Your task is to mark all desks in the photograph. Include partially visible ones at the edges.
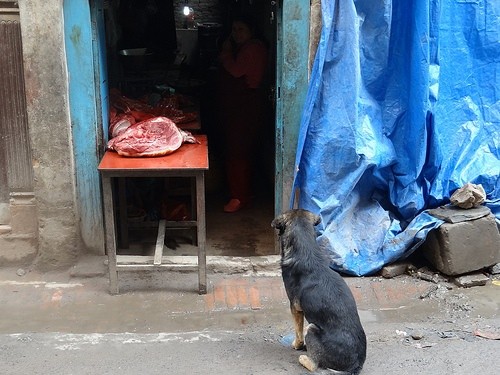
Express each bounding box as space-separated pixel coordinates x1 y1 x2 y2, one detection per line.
97 134 210 295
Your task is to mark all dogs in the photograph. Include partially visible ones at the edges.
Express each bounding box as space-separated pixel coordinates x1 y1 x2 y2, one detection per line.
270 209 367 375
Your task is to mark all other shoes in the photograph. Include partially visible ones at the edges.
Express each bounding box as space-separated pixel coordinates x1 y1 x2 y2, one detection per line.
224 197 244 212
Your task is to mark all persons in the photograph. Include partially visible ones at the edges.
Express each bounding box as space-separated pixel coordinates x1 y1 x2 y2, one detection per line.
218 15 269 213
